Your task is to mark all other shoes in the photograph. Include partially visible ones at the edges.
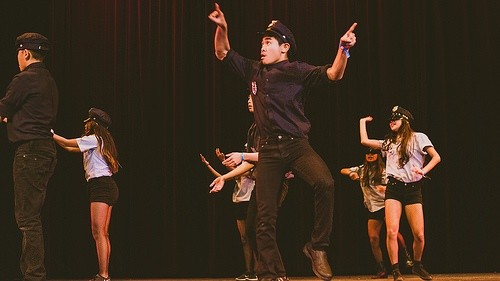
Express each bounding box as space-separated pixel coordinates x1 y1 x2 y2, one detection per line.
261 276 290 281
393 270 403 281
371 272 388 279
236 272 259 281
302 243 333 281
406 261 414 274
88 273 111 281
412 263 433 280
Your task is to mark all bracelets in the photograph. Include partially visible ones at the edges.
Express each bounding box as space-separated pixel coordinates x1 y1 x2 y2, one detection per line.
51 132 54 136
241 152 244 160
349 171 353 176
339 44 350 58
421 170 424 176
205 163 209 166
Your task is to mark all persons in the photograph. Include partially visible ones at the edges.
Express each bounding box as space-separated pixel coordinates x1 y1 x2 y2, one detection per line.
208 3 358 281
199 106 441 281
51 106 121 281
0 33 58 281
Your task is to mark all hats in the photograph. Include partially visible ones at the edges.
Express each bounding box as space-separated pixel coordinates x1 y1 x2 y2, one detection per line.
390 105 414 121
15 32 49 51
257 19 296 47
84 107 112 130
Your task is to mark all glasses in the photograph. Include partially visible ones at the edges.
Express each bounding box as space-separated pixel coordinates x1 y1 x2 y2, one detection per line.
365 149 381 154
